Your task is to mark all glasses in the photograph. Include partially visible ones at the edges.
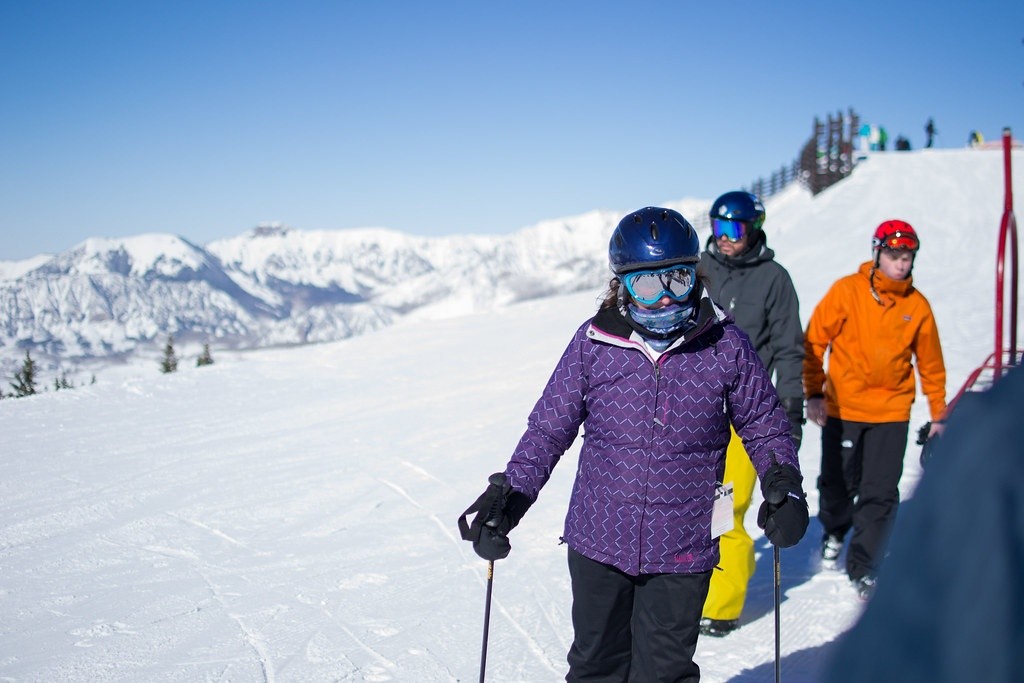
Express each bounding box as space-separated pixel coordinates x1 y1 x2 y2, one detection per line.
883 231 919 250
616 264 696 308
711 217 755 243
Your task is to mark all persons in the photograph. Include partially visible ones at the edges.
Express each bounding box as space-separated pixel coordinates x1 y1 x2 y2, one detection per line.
694 191 807 637
803 219 947 602
461 206 810 683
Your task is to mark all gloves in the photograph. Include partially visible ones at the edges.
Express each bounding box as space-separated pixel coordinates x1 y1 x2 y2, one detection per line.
757 463 809 548
780 398 805 451
916 422 941 468
458 483 531 560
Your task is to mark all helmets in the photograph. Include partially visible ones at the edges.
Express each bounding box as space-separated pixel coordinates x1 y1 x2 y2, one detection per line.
872 220 919 252
709 191 765 235
609 207 699 274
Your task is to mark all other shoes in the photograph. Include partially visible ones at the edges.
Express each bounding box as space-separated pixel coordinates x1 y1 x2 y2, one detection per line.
698 617 739 637
822 535 843 569
848 571 881 601
